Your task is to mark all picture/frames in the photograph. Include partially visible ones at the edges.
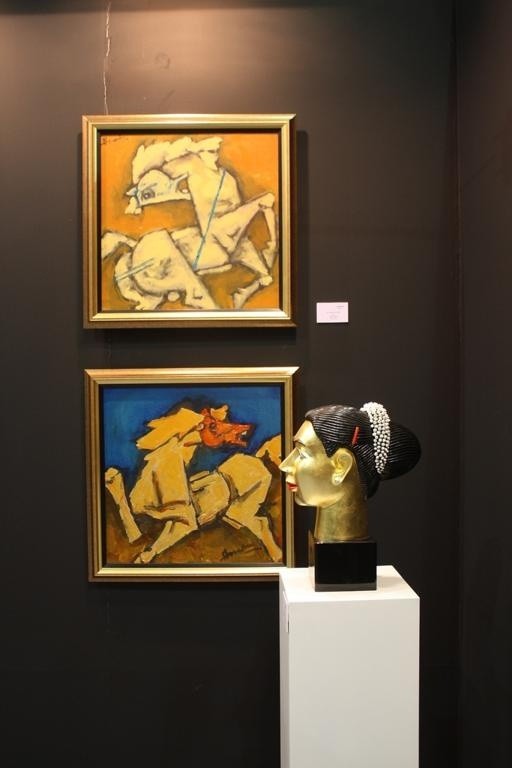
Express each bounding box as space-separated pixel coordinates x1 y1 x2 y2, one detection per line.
83 368 296 584
80 113 298 333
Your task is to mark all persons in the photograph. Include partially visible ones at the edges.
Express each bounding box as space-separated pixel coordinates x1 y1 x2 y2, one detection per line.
276 396 423 542
